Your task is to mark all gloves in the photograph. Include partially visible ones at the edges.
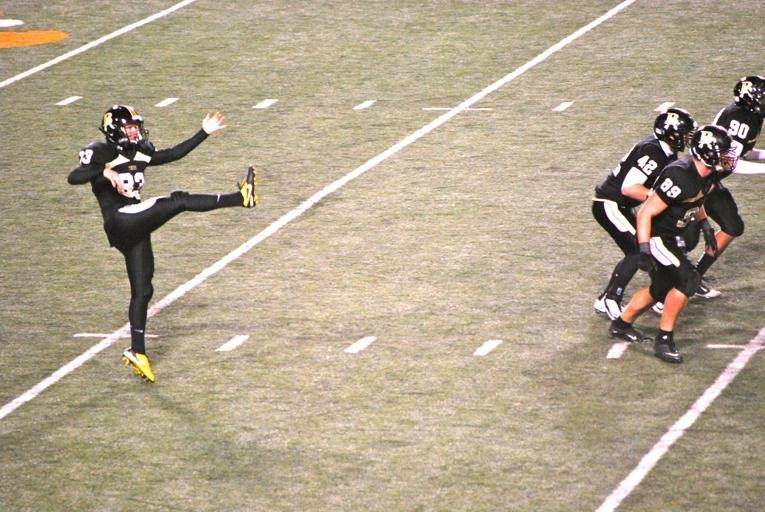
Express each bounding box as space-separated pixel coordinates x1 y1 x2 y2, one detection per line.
702 227 718 258
638 252 659 274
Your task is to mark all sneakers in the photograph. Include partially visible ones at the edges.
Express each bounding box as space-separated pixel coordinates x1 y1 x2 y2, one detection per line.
238 164 259 208
653 336 683 363
607 319 653 343
648 300 665 316
593 292 621 321
121 346 154 384
694 283 722 299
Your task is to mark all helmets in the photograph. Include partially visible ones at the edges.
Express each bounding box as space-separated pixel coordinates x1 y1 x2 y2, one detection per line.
654 108 700 152
734 75 765 121
99 105 149 154
690 124 738 173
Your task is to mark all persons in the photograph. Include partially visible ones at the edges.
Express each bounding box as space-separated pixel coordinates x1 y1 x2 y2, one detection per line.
593 77 764 363
68 104 257 382
